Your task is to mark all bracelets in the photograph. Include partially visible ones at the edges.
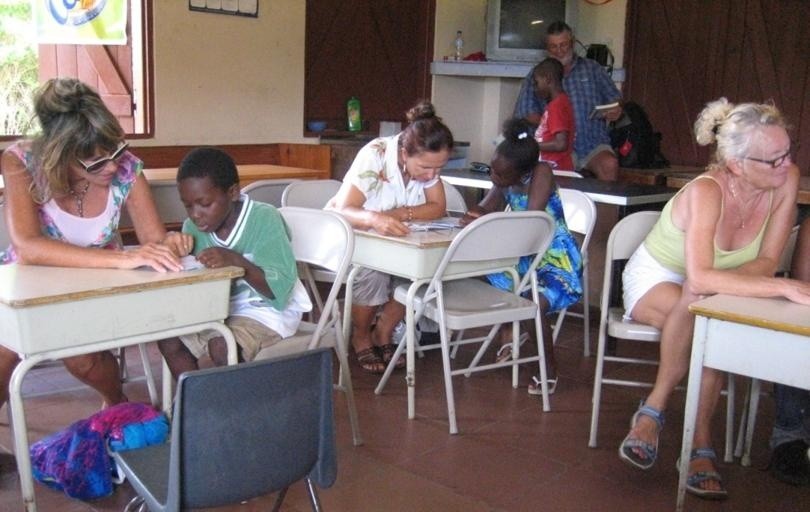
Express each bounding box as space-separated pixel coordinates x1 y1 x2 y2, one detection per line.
403 205 413 221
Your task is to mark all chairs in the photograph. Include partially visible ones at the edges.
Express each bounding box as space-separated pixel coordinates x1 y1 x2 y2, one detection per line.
449 186 596 388
588 211 737 462
373 209 558 435
282 178 424 359
237 177 316 323
106 346 338 511
250 205 364 447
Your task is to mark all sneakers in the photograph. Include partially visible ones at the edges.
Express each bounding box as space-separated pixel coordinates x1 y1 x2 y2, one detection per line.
770 439 809 486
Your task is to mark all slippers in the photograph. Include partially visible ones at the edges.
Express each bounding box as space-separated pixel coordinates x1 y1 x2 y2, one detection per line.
528 374 558 395
675 448 727 500
497 333 528 363
351 342 406 373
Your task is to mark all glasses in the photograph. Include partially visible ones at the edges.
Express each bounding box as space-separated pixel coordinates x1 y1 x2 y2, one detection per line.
74 140 128 172
743 141 798 168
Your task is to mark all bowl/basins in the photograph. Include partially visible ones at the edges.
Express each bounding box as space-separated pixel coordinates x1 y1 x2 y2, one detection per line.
307 121 328 134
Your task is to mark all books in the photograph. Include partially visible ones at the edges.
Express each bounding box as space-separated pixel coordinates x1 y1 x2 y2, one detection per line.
589 102 619 121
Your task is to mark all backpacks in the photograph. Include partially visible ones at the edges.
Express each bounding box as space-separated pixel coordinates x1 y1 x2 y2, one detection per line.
608 101 670 169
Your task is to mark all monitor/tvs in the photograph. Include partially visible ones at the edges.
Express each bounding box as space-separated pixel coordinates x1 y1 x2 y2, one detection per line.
486 0 577 65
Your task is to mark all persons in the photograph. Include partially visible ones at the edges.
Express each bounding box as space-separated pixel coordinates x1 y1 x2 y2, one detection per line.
1 79 195 411
322 100 452 374
619 96 809 499
512 20 624 182
769 217 810 486
458 118 583 391
157 147 313 382
533 57 574 171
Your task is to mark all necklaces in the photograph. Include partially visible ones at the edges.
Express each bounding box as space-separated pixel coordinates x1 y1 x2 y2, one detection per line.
65 180 90 217
729 180 758 229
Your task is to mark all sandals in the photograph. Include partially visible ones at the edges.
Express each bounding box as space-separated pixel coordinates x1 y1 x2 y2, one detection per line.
620 402 665 470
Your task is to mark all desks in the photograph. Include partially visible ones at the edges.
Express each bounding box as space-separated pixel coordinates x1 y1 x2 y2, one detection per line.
320 138 471 181
1 243 245 512
339 217 520 420
675 295 810 512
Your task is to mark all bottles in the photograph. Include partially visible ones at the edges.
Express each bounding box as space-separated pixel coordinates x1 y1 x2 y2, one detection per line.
347 95 362 132
454 30 464 61
